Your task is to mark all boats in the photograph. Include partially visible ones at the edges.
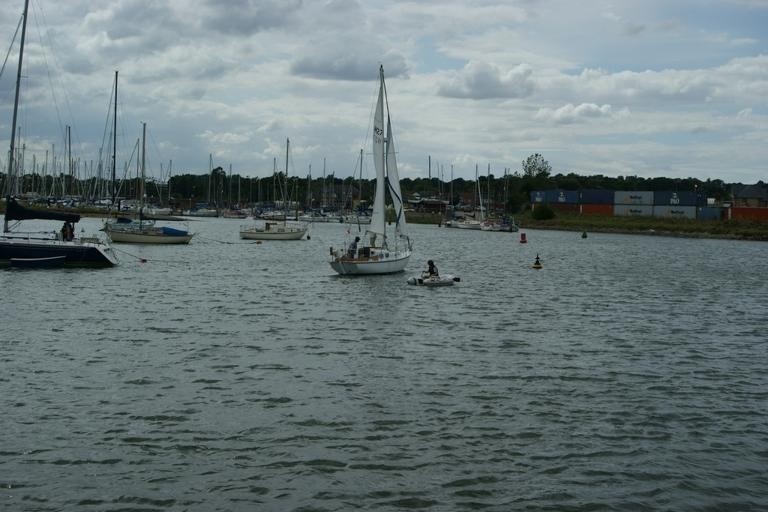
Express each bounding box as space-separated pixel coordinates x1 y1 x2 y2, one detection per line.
403 270 461 290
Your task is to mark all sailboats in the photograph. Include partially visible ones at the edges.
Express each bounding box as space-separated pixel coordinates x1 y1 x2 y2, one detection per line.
99 121 194 244
0 0 123 268
236 136 309 240
194 153 220 218
249 137 520 242
0 72 187 221
324 62 416 277
222 174 248 220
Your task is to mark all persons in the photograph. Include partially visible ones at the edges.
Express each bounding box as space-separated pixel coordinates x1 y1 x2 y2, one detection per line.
347 235 361 259
421 258 438 278
